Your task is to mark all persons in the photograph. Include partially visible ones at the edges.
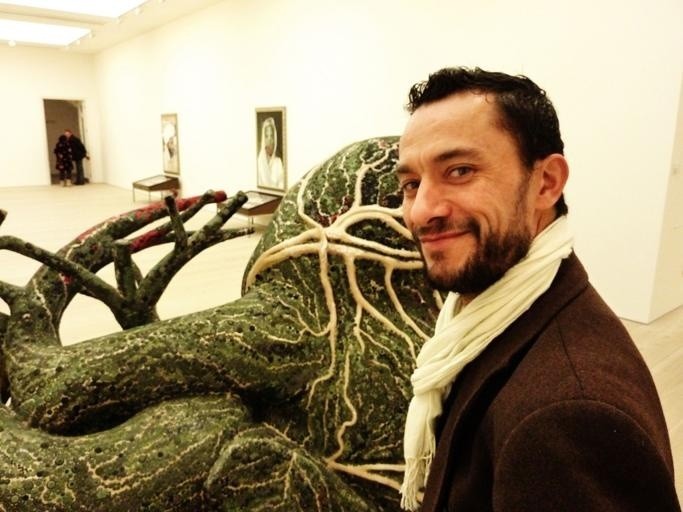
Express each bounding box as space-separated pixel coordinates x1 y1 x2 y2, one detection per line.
64 129 87 185
393 67 681 512
53 133 74 186
256 117 284 191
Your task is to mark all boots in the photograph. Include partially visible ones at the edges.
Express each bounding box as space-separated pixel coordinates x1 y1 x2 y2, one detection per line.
59 179 72 187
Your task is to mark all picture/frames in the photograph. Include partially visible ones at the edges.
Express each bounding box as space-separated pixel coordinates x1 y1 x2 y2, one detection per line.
252 106 288 194
159 113 181 176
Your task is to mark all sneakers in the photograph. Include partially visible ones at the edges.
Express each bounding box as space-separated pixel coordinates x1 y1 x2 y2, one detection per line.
73 180 83 185
